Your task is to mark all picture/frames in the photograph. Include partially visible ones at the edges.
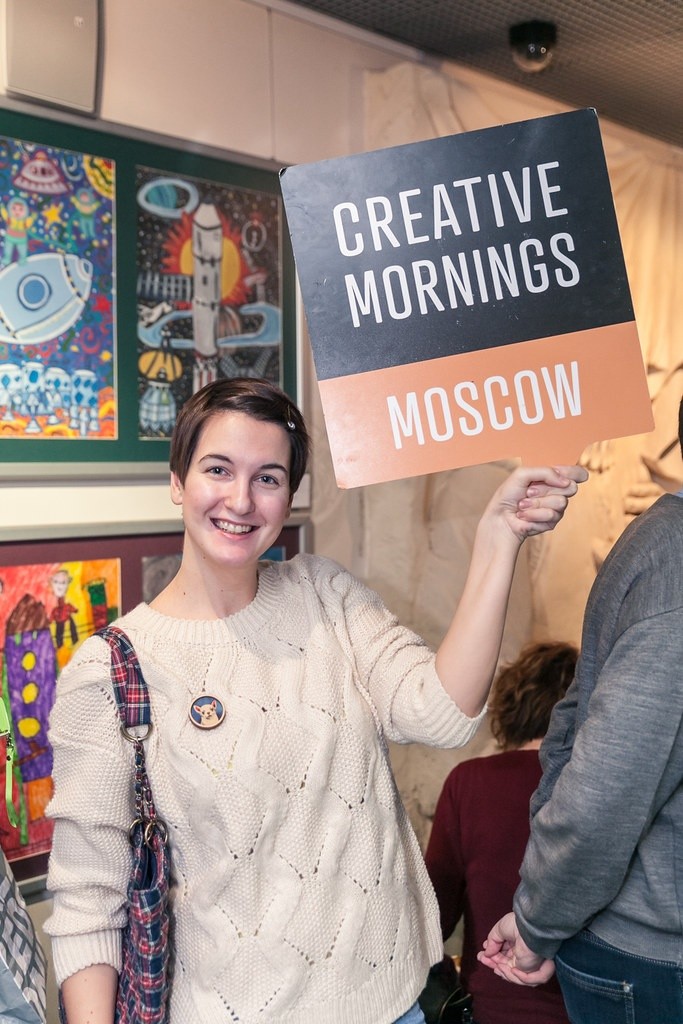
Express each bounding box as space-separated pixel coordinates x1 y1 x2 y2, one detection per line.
0 94 306 484
0 511 315 899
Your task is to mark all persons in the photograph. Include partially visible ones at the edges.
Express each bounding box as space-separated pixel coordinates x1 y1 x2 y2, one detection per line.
477 392 683 1024
425 640 582 1024
43 376 588 1024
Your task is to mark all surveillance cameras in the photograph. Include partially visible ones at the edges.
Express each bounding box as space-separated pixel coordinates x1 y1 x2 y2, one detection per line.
507 20 557 72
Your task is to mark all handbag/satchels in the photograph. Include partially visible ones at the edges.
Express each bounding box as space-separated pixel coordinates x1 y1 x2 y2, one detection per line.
0 848 47 1024
57 626 171 1023
